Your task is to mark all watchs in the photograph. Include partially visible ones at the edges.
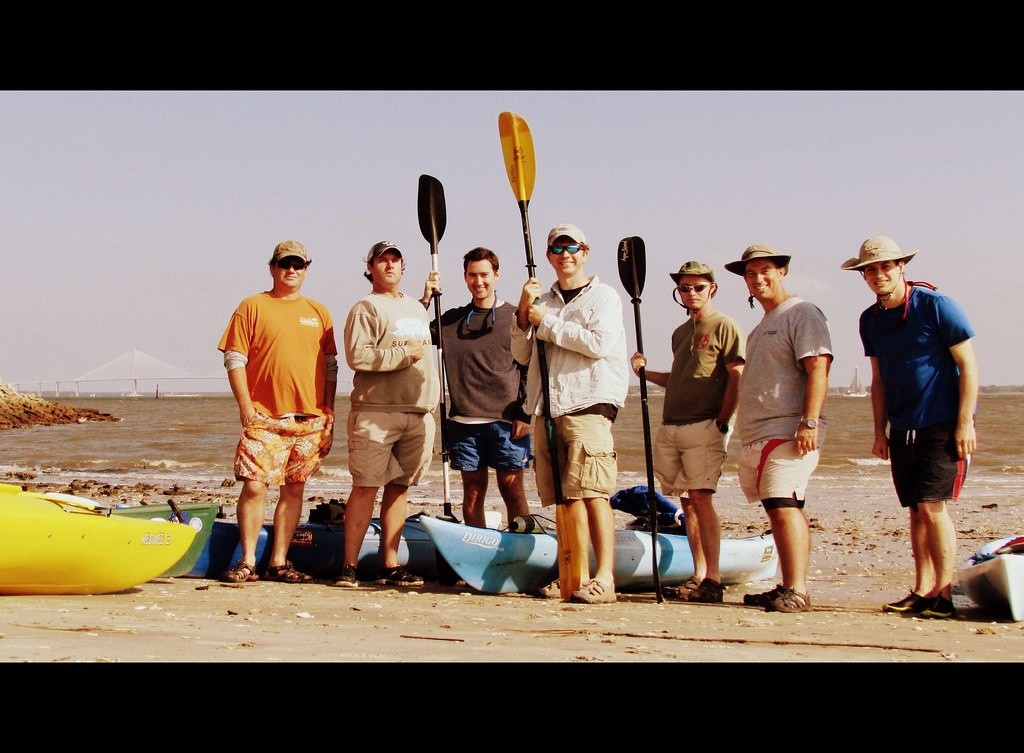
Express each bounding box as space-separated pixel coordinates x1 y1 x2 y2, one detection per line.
801 418 819 428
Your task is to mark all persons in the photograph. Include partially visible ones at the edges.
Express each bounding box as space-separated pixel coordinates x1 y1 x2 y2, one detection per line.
216 240 338 583
419 247 533 529
330 242 442 587
840 236 978 616
724 245 835 611
510 224 629 604
629 261 746 603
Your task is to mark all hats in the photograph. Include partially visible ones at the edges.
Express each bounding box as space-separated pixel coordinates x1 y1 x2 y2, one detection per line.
840 235 918 270
669 261 718 299
367 240 403 261
723 245 791 277
547 224 586 248
273 240 307 263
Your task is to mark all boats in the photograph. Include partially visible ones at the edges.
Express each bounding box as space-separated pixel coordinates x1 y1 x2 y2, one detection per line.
1 483 202 595
419 514 779 595
184 518 442 579
95 503 220 579
959 535 1024 622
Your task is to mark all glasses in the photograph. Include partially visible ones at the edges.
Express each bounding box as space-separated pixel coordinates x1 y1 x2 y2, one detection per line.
679 282 713 293
274 259 307 270
467 307 495 335
547 245 585 254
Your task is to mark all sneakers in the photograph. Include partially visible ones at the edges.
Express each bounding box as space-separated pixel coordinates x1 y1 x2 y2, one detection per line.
921 591 957 618
882 589 928 614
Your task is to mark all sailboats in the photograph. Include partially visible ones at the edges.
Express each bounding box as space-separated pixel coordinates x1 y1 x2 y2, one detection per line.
842 366 868 398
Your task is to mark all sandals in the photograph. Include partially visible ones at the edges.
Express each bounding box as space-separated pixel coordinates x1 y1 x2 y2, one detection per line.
682 578 726 604
227 560 259 582
376 565 424 586
263 560 313 583
662 575 703 601
335 563 359 588
539 578 561 599
743 584 787 607
572 579 616 604
765 587 813 612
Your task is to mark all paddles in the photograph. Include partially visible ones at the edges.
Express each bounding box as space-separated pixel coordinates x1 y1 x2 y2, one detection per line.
417 174 460 525
498 112 582 599
617 236 664 605
